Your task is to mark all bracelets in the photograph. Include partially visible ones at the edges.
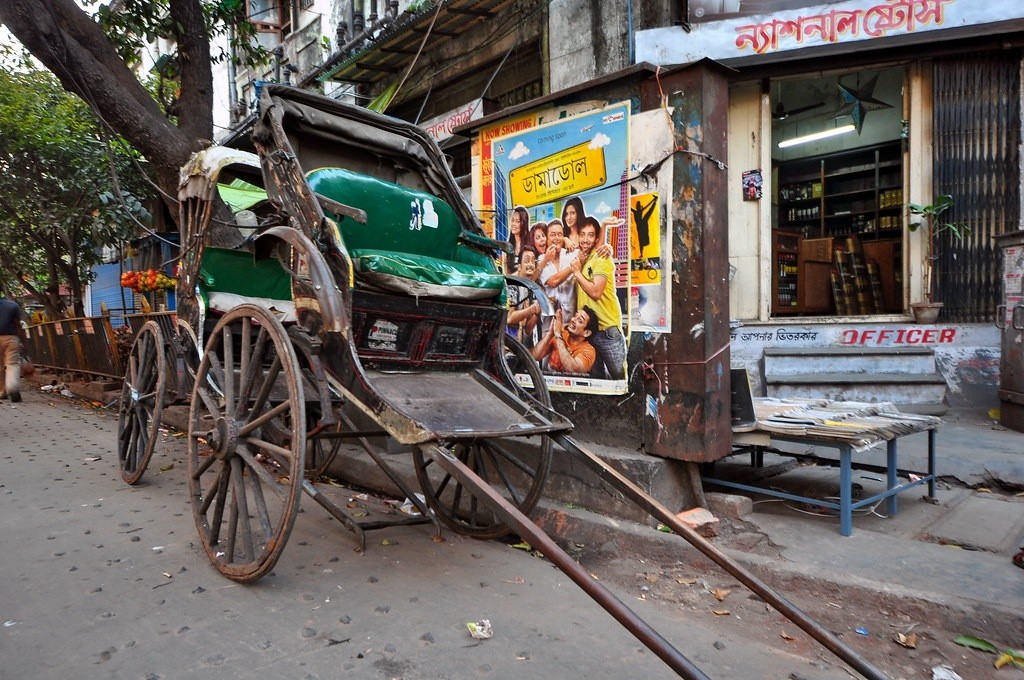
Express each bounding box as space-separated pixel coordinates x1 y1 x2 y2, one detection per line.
537 266 542 272
555 334 562 340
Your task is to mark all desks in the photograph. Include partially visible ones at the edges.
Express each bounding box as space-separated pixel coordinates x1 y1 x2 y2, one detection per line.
194 242 300 323
698 404 943 537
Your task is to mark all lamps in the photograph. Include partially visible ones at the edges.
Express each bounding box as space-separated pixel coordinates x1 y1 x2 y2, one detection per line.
774 78 856 151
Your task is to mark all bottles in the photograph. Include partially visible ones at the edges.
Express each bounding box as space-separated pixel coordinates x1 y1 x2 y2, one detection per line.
776 180 905 316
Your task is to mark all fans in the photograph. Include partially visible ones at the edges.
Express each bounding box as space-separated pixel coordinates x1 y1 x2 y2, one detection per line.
768 79 826 122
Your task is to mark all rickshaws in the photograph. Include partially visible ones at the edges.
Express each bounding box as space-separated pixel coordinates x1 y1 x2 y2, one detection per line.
117 82 890 680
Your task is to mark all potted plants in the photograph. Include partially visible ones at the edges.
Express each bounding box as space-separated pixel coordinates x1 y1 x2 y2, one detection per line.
904 194 973 325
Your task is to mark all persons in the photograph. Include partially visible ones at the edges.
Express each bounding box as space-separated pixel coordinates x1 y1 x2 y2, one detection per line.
0 297 24 402
495 197 626 379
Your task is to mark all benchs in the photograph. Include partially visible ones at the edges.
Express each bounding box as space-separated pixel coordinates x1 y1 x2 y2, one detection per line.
303 164 506 306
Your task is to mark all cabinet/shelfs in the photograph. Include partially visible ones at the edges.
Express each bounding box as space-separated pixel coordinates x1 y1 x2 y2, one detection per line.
777 138 904 239
768 227 904 314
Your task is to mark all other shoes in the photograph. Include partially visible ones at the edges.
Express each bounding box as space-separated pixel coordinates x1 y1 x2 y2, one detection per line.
10 391 23 402
0 393 8 400
